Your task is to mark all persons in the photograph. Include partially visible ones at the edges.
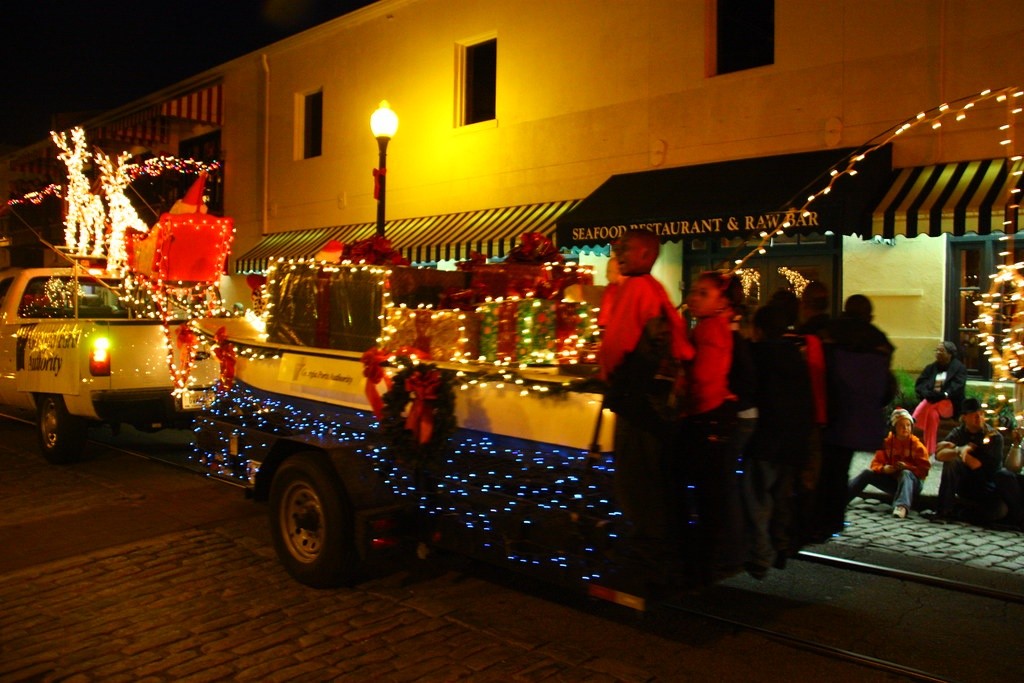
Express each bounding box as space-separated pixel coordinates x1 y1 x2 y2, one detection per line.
913 341 967 456
848 407 930 517
934 399 1005 523
596 230 895 592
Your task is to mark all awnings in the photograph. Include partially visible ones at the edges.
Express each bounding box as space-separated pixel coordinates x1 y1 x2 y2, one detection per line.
11 127 120 176
100 106 171 144
236 199 581 275
555 143 894 249
145 77 224 125
862 157 1024 240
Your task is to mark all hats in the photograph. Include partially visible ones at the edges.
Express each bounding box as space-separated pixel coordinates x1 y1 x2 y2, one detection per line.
891 407 915 426
956 398 981 414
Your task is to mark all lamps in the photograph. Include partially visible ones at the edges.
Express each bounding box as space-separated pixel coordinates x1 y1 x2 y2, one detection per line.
872 236 896 247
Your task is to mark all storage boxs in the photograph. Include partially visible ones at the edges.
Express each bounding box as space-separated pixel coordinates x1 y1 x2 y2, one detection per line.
390 296 591 366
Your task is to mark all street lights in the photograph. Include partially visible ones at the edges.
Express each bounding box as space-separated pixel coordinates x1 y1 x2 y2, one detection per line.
369 100 399 234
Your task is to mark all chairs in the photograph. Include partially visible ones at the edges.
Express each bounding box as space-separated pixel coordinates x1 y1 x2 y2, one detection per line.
79 296 113 317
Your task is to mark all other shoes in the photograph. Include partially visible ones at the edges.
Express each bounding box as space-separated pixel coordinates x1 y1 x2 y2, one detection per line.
935 501 956 521
892 506 907 518
976 507 999 531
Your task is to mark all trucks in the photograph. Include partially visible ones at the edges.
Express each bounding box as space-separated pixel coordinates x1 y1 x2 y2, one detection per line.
0 267 262 464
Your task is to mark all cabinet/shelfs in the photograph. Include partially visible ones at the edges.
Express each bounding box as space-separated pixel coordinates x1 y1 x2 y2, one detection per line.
958 288 981 372
